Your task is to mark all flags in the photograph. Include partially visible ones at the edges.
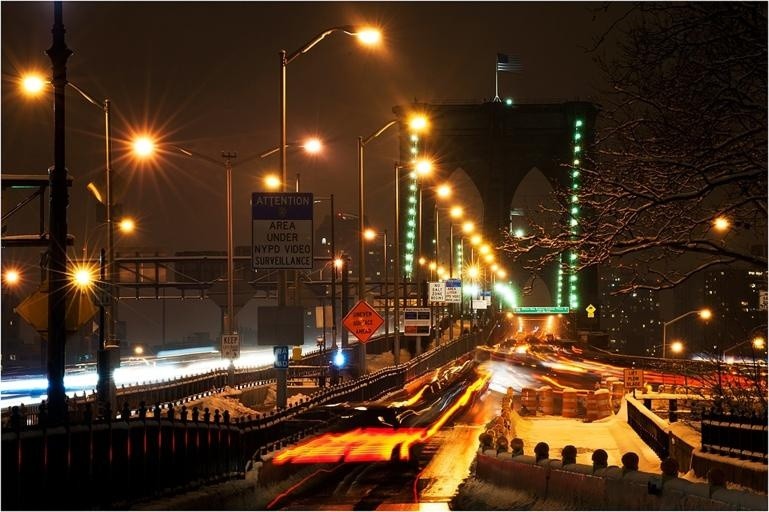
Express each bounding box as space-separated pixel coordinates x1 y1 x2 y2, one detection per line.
496 53 526 74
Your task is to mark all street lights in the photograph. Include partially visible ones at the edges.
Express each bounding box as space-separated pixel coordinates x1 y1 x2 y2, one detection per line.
662 309 711 358
136 135 325 383
21 74 118 424
722 337 764 362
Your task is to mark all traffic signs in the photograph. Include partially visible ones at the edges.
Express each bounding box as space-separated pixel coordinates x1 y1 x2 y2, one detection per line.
252 190 314 270
513 306 570 314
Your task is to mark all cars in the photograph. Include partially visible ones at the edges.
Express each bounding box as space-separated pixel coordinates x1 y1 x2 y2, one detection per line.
496 324 580 357
